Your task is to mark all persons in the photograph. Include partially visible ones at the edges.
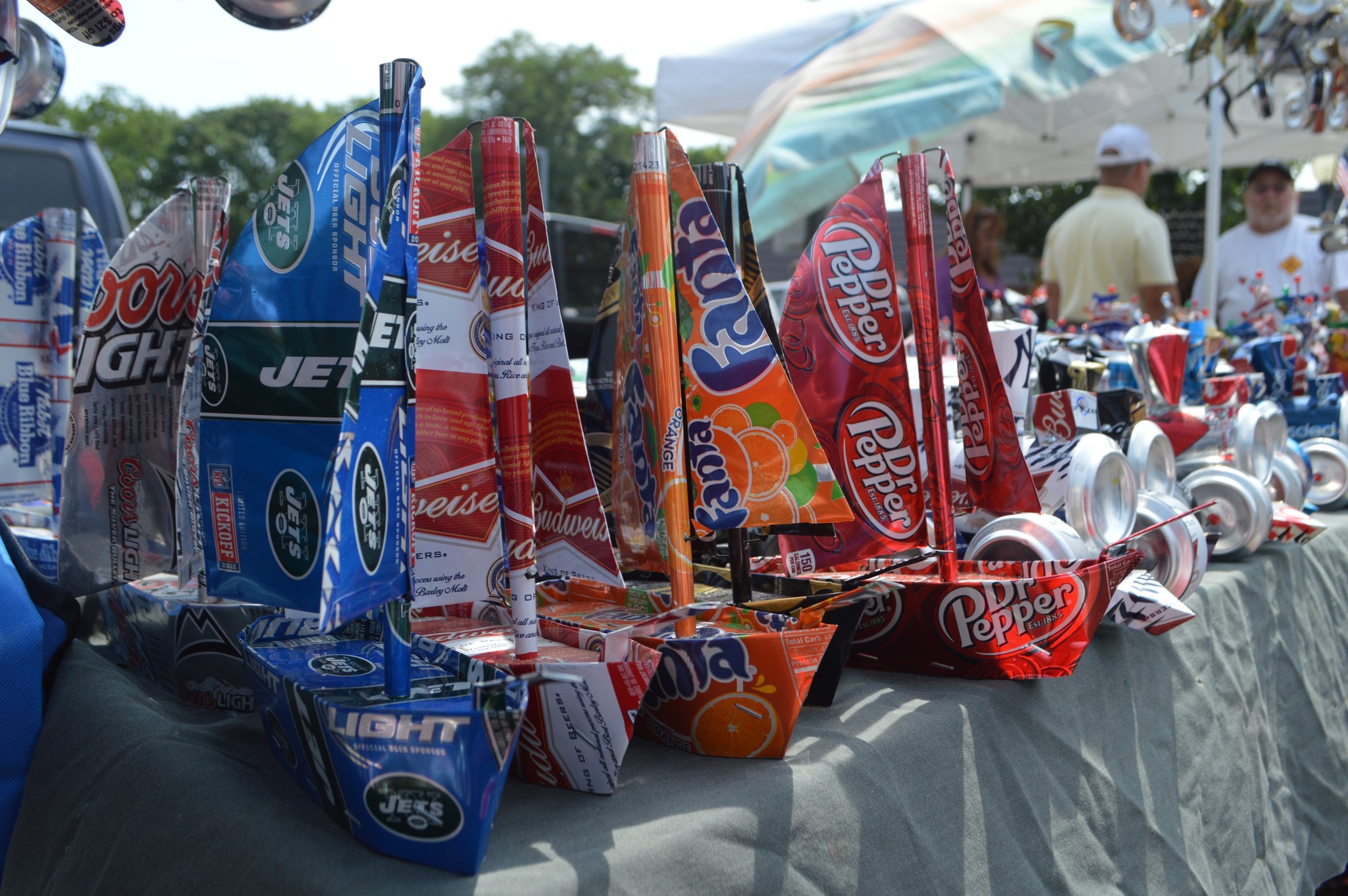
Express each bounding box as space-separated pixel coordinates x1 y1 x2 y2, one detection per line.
959 202 1007 321
1189 159 1347 334
1039 124 1180 328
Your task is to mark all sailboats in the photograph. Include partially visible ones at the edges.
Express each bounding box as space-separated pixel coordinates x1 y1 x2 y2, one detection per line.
10 55 1140 880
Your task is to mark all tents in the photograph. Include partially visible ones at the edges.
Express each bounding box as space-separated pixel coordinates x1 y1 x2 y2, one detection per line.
653 3 1347 183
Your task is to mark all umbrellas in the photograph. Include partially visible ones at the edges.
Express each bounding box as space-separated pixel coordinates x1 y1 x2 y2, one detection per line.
724 2 1222 244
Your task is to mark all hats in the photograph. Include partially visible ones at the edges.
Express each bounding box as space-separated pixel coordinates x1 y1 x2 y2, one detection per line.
1095 124 1161 167
1247 157 1293 181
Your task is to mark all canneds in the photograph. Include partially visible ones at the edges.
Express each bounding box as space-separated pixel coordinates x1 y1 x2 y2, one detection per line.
915 328 1348 611
